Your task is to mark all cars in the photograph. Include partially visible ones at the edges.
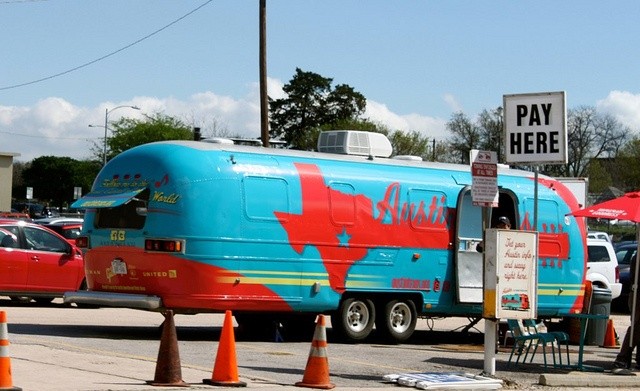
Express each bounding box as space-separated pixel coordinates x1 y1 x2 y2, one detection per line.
34 217 84 224
0 218 94 308
30 205 45 217
614 242 638 313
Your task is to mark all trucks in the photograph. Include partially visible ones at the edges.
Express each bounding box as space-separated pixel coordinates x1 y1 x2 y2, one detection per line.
64 129 588 340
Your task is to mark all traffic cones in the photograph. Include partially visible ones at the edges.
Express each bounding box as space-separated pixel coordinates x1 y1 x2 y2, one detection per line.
599 318 620 348
203 309 247 386
146 309 191 386
295 313 336 388
0 310 22 390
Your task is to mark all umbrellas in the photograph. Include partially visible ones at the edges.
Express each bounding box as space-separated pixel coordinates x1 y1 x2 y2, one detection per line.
564 192 640 222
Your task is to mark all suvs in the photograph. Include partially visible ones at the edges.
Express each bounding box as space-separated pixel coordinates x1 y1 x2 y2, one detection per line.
586 238 623 298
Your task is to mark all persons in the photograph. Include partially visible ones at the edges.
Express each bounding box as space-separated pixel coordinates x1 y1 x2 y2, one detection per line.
475 217 511 253
615 254 640 370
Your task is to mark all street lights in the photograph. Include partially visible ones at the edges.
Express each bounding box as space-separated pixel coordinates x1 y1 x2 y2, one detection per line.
105 105 140 164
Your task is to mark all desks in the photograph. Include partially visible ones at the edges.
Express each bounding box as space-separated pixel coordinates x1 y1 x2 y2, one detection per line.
556 314 610 371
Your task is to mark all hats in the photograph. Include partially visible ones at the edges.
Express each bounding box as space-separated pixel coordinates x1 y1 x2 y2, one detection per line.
498 216 510 224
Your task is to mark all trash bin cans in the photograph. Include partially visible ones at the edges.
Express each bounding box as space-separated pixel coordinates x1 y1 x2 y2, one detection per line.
585 289 612 346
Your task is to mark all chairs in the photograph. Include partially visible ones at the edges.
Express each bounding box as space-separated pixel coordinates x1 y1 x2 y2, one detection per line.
507 319 556 370
523 319 571 367
